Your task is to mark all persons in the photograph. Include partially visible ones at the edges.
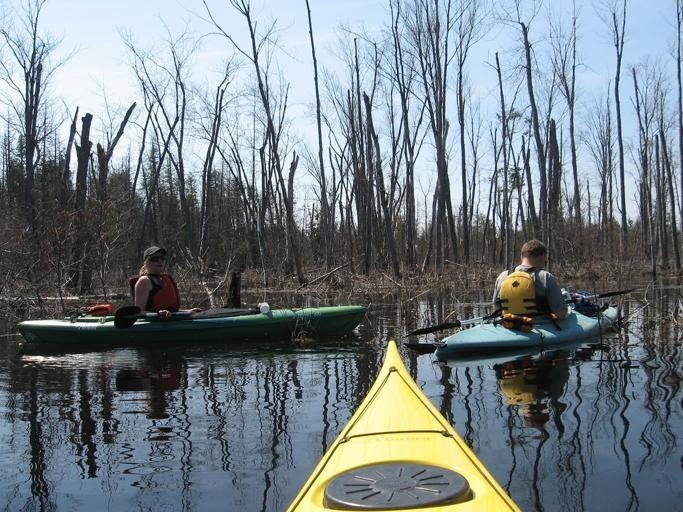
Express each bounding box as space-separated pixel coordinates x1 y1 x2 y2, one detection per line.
493 354 562 429
134 246 201 320
491 239 567 322
134 341 182 419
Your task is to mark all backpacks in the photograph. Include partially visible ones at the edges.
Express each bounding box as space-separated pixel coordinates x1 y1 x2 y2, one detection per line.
500 267 544 317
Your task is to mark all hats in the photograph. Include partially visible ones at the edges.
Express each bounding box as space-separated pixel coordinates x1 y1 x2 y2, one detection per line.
143 246 166 262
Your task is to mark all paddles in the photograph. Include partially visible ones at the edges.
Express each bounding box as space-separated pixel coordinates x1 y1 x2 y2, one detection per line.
115 306 196 329
403 289 638 337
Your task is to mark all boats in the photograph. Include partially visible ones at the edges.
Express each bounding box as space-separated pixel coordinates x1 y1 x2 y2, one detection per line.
15 303 368 356
286 339 528 512
433 297 621 362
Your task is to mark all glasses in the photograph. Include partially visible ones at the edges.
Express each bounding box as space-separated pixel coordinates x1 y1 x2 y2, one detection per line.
148 255 166 262
